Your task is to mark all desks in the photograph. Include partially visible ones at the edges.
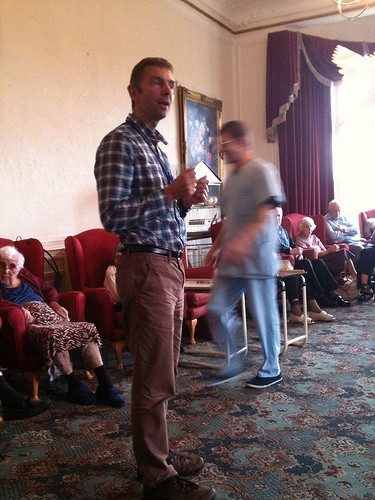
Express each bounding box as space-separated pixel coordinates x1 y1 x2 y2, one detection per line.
247 270 309 355
179 280 247 368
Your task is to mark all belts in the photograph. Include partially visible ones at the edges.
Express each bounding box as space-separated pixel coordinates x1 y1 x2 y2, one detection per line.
124 244 182 258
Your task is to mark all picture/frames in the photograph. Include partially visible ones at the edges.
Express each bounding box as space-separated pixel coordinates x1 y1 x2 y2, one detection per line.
176 87 224 208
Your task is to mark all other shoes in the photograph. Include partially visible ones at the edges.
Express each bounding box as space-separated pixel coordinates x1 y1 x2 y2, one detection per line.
311 310 336 323
291 313 314 325
246 373 283 388
358 285 370 300
202 367 246 386
2 394 53 420
68 385 97 404
97 384 125 407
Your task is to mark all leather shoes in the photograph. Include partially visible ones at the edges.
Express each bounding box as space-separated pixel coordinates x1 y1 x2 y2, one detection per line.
318 297 338 308
329 295 351 307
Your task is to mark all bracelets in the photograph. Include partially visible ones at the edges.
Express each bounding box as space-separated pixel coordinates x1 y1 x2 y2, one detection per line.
177 198 190 214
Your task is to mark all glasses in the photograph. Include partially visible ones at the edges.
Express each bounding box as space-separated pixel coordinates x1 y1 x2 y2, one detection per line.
0 261 17 271
220 137 240 147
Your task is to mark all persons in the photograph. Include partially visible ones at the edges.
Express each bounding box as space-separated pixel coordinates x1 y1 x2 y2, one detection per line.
93 59 216 500
0 246 125 407
205 120 283 387
276 200 375 324
102 241 183 354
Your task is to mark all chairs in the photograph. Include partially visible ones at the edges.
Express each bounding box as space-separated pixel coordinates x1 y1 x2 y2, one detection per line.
0 228 219 401
280 209 375 267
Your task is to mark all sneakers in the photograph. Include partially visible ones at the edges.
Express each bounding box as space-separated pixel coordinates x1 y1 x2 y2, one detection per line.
136 450 204 481
142 475 216 500
